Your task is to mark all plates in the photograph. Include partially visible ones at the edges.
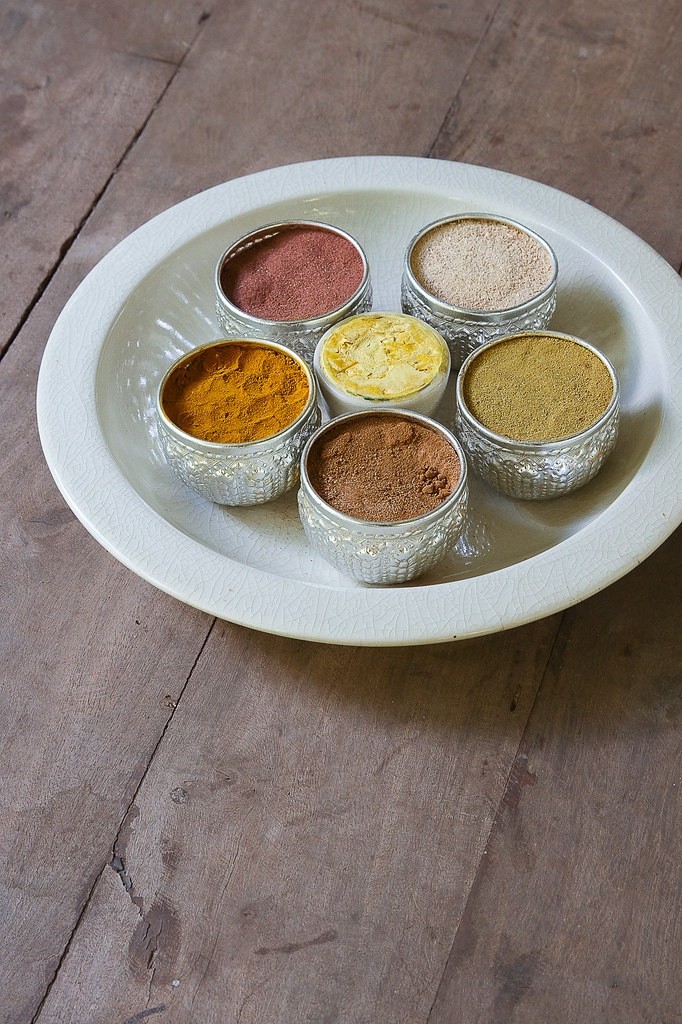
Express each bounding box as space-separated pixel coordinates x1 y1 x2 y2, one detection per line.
34 154 682 647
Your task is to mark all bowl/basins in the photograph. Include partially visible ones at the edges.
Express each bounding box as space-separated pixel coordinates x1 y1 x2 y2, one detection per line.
159 335 322 507
453 329 619 501
213 219 374 375
401 212 560 371
297 407 475 586
313 311 452 437
